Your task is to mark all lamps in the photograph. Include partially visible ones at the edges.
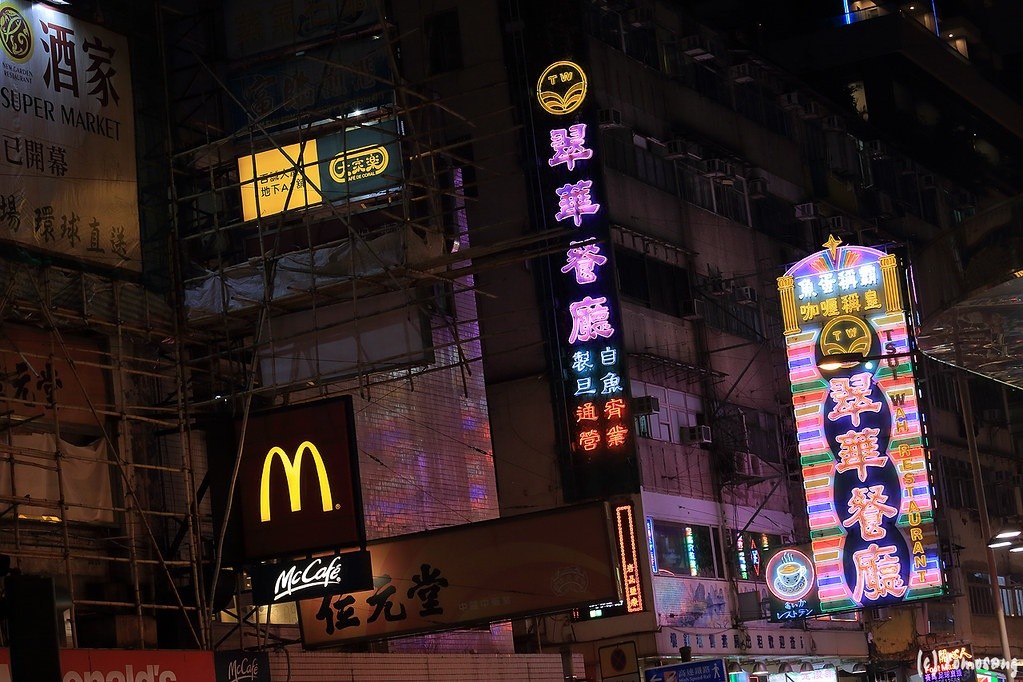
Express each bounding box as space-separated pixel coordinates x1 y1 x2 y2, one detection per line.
987 494 1023 552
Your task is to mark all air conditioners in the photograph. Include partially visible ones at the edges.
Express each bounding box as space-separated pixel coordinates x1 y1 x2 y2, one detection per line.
683 425 712 445
731 450 764 481
